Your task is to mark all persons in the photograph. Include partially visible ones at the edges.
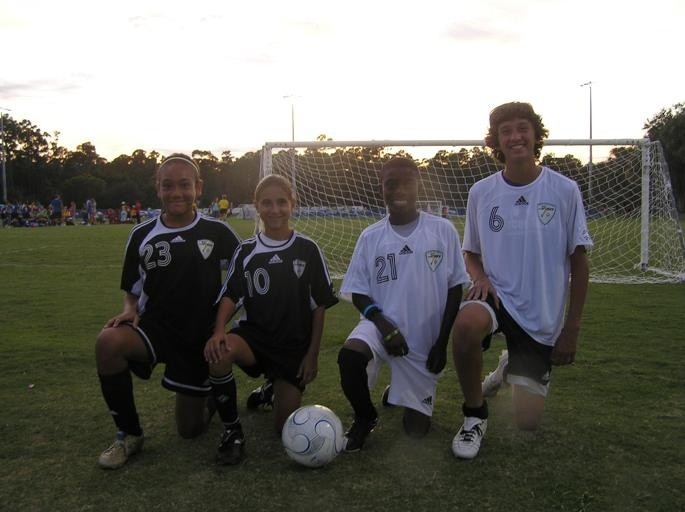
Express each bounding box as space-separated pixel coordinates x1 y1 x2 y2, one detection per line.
0 194 156 228
337 157 472 453
451 102 594 459
94 153 244 470
208 194 234 222
204 175 339 466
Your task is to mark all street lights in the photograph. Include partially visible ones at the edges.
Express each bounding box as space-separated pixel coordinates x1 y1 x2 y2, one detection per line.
283 91 304 209
578 79 595 211
0 106 13 205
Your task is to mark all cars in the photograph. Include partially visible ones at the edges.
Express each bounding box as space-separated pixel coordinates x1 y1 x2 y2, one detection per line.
139 208 161 217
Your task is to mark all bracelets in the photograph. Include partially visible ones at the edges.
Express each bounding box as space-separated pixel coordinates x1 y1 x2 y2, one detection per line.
383 328 400 343
363 304 378 317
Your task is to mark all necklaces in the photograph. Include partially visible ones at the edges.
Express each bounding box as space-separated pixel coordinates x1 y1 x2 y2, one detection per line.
257 232 293 248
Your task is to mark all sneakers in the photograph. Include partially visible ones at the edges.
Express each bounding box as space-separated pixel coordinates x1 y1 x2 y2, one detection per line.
99 428 144 470
452 416 488 460
247 379 275 411
217 430 246 466
482 348 509 397
342 407 378 453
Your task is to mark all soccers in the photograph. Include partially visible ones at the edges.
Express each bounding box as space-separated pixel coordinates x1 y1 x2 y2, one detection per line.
282 404 345 468
